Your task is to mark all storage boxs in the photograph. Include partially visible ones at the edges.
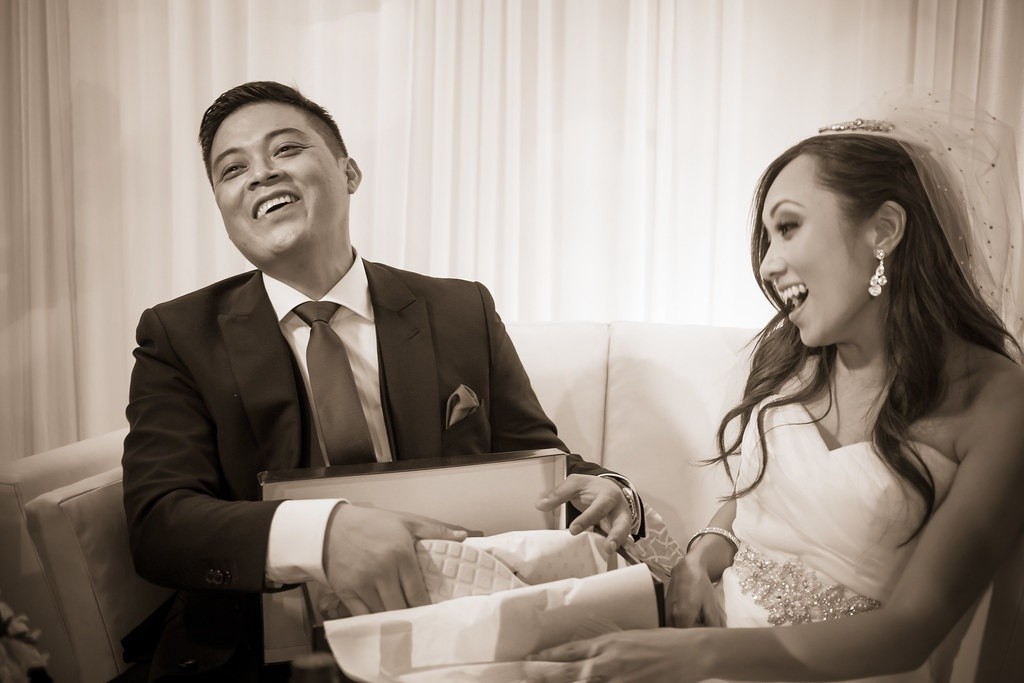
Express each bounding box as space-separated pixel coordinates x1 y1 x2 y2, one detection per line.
256 446 667 682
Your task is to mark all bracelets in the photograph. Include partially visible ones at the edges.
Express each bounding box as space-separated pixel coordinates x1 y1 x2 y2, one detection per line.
684 526 741 552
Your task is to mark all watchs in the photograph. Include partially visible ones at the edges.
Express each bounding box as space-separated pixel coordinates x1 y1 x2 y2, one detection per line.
612 476 640 522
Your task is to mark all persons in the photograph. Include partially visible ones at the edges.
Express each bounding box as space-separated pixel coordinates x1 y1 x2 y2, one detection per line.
524 88 1024 683
121 79 644 683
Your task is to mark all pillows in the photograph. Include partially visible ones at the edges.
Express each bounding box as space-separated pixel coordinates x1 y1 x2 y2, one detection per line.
501 321 610 467
22 464 172 682
601 323 767 553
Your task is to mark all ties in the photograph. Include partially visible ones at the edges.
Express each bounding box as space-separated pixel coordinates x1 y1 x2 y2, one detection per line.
292 301 378 467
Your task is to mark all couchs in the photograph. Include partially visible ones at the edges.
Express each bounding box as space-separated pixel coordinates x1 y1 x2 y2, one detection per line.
0 421 1022 682
